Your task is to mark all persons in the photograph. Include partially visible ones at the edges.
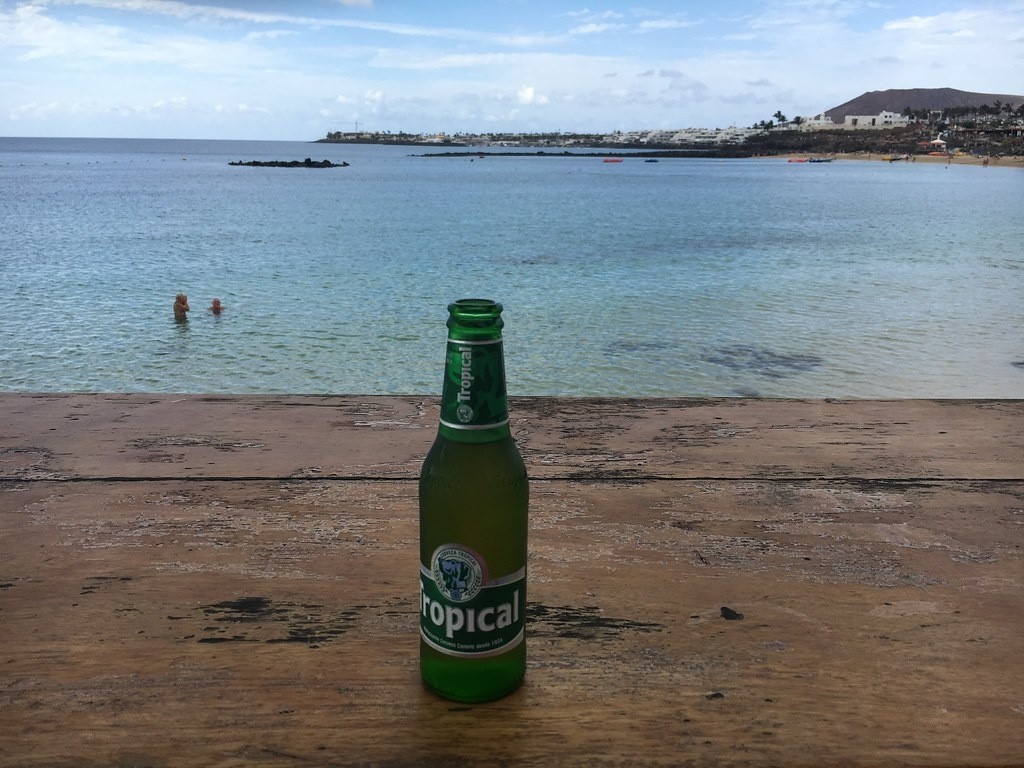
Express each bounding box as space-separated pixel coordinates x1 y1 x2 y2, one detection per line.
208 299 224 310
174 293 190 320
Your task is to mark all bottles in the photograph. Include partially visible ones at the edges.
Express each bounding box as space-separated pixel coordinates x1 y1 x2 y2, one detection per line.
419 298 529 703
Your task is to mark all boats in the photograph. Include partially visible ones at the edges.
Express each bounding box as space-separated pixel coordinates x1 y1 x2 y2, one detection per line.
809 156 833 163
645 159 659 163
883 154 909 161
603 158 624 162
787 157 809 162
